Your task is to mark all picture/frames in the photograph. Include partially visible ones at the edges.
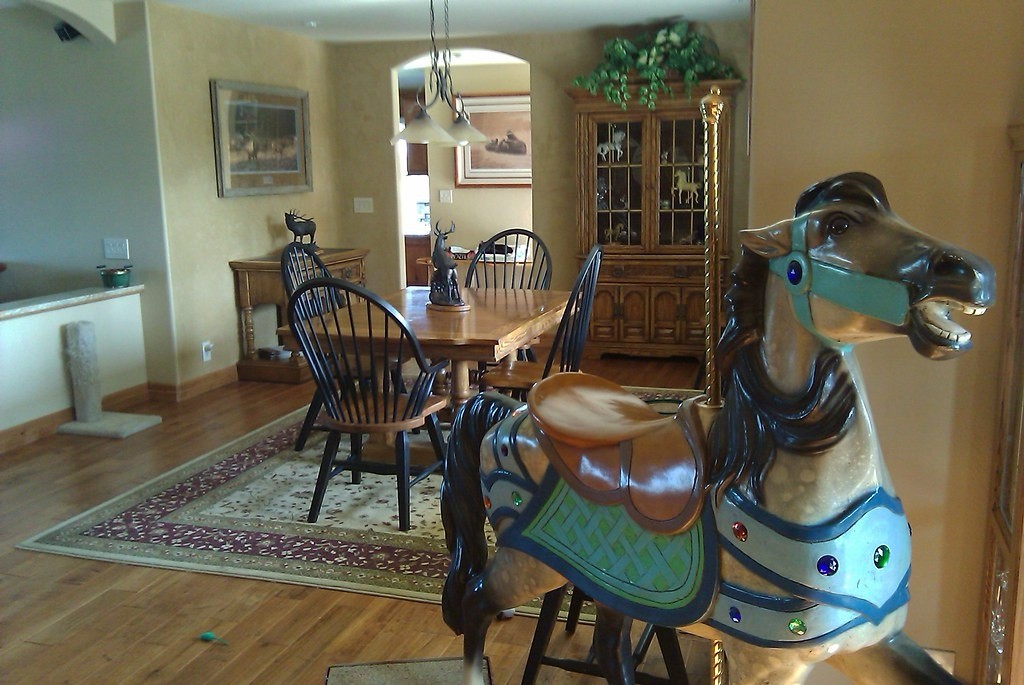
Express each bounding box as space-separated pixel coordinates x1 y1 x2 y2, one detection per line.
449 92 532 188
210 78 313 199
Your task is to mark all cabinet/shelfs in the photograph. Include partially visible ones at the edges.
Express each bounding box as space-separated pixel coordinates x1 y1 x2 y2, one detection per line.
567 72 741 360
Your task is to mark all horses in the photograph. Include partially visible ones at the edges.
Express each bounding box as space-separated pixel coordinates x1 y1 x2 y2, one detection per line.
597 132 626 161
604 223 624 240
670 169 702 204
442 171 995 684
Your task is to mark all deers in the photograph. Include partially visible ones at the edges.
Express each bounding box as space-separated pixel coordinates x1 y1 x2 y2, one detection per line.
432 220 465 306
285 209 316 245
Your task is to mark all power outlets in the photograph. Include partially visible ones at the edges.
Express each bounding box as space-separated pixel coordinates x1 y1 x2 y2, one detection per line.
202 341 212 362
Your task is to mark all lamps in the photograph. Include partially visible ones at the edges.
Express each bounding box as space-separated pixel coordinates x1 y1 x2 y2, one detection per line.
390 0 489 145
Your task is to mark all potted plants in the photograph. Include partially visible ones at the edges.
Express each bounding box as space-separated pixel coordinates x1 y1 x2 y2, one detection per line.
584 20 738 110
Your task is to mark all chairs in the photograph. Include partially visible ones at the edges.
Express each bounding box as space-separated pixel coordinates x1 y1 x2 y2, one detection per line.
465 229 553 372
287 278 449 532
283 241 421 451
485 246 601 402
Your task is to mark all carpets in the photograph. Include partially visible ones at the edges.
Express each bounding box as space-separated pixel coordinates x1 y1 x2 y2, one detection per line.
13 381 714 623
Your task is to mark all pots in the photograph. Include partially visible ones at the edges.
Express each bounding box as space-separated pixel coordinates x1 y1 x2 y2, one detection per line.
96 265 133 288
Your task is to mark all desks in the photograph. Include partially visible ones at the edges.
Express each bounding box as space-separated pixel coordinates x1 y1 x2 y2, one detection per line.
229 247 369 382
416 255 535 289
